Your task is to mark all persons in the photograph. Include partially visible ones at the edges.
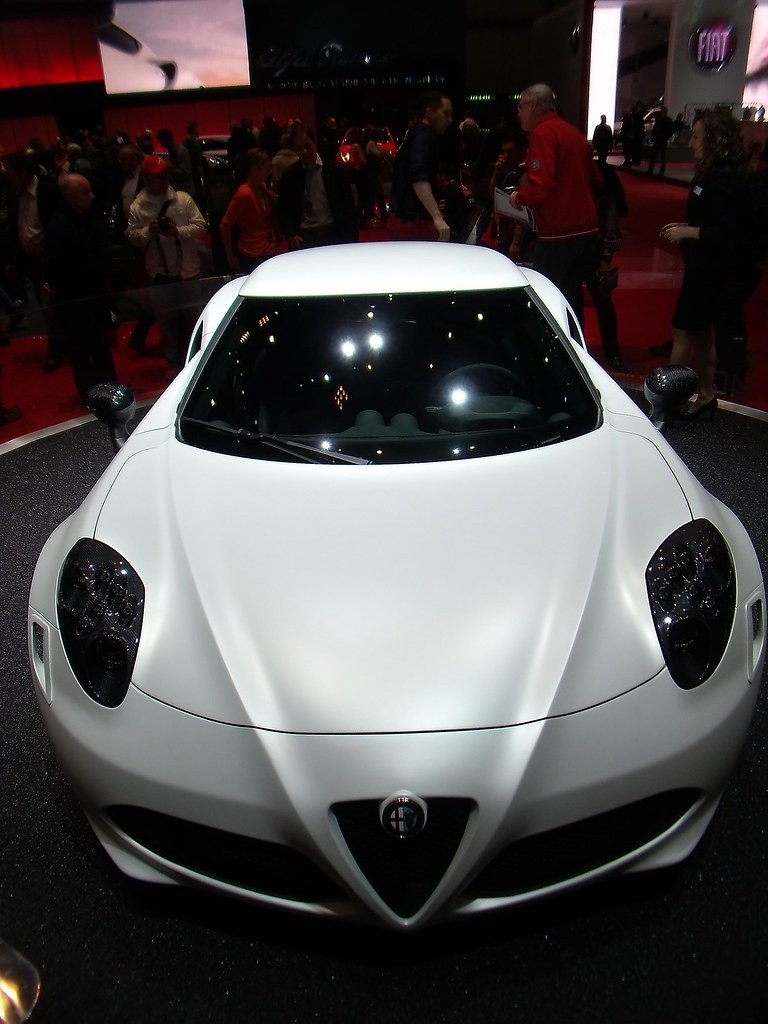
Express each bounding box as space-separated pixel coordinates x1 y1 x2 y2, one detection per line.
0 82 768 426
125 156 207 379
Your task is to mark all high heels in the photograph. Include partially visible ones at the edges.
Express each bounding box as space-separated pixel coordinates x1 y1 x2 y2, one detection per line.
678 398 719 420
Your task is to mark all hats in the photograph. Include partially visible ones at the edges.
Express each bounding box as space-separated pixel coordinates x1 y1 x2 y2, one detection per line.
140 154 169 178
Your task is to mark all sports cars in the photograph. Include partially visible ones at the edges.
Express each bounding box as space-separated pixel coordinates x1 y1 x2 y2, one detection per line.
22 238 768 932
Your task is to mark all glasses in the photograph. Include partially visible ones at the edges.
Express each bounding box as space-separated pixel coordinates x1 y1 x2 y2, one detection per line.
517 100 532 113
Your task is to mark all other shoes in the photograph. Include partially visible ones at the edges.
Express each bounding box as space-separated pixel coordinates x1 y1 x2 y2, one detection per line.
711 371 740 395
370 214 376 218
128 337 149 355
42 357 64 370
650 338 712 358
0 407 20 425
162 348 186 368
382 210 387 218
598 356 625 374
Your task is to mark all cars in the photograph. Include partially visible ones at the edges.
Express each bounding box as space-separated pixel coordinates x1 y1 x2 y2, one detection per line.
613 106 662 141
334 123 399 173
151 131 235 184
